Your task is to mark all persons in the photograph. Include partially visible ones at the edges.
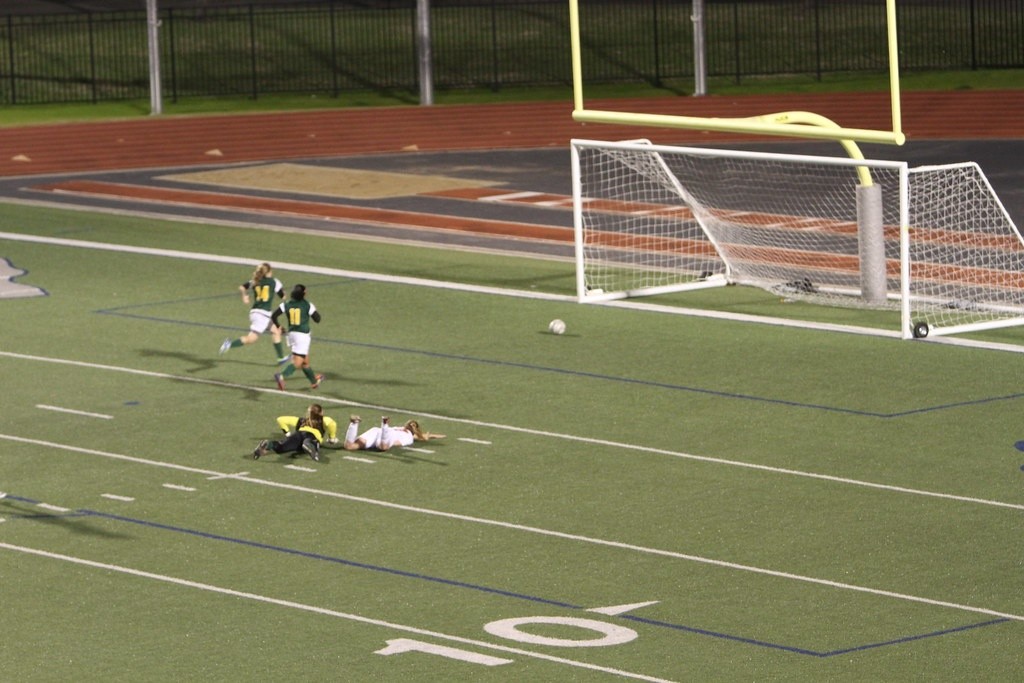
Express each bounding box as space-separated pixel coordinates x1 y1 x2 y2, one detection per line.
254 404 339 461
271 285 324 391
219 263 292 364
344 415 447 451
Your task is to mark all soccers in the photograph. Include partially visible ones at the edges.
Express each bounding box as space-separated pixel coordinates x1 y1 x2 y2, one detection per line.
549 319 566 334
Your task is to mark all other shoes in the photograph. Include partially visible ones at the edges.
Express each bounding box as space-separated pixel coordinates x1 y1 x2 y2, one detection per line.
253 439 269 460
302 443 320 462
275 373 286 391
218 336 231 356
311 374 324 388
350 416 361 424
277 355 291 363
382 416 389 424
